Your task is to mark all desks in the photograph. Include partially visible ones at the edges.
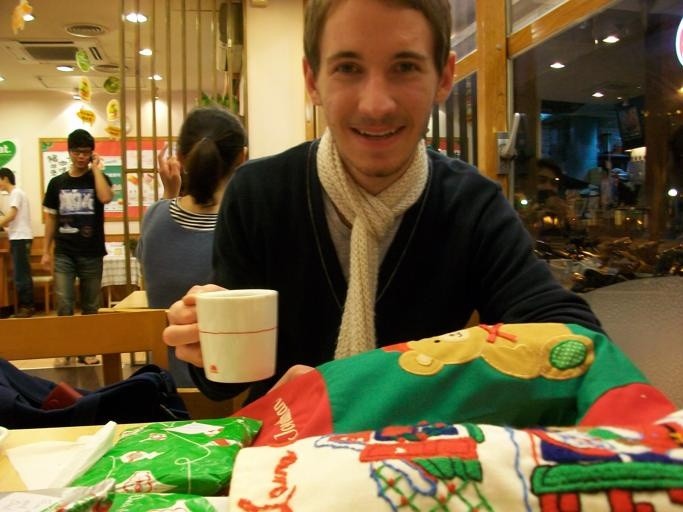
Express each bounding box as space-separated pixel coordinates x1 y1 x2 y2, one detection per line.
102 242 148 308
0 420 155 491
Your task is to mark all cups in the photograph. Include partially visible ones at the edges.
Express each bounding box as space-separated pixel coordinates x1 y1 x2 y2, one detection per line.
187 287 278 385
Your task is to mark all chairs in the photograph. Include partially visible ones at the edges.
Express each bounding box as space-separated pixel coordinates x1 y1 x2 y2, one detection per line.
1 309 168 371
0 237 55 315
96 309 251 420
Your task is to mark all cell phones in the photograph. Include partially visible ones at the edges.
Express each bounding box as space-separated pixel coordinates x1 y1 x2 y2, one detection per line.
90 151 92 162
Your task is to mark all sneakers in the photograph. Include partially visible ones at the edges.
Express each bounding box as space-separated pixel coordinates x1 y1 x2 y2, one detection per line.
53 355 99 368
7 305 37 319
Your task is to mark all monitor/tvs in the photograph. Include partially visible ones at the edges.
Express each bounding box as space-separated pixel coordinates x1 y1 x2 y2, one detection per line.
615 101 645 150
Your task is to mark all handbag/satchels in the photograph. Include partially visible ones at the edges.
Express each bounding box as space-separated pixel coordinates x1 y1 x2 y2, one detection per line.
0 357 192 429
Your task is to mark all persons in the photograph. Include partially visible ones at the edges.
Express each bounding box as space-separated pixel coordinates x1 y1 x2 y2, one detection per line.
134 105 251 389
157 0 614 408
0 166 37 318
522 106 683 237
41 128 114 316
